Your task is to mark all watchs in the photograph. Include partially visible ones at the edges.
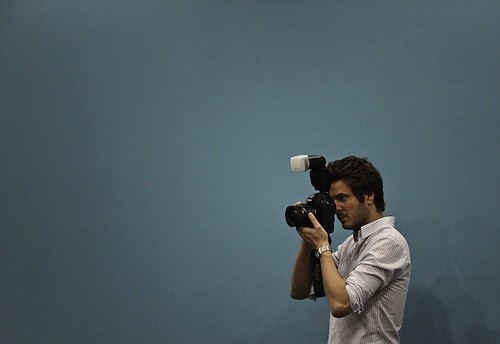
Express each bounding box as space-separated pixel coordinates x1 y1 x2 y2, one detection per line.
314 246 333 258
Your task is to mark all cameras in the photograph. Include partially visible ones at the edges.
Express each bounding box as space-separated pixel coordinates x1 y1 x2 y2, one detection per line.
285 154 337 233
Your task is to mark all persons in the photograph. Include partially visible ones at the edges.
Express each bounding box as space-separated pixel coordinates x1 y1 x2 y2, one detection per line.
289 155 410 344
